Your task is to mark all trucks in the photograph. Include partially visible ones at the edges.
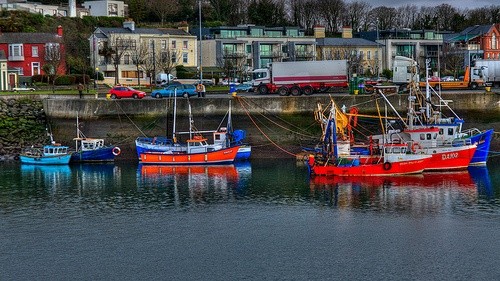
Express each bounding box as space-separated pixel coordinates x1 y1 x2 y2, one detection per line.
252 59 351 96
360 56 420 94
419 65 487 91
156 74 177 83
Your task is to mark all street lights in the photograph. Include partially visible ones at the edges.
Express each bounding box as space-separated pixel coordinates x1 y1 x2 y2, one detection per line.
95 67 99 87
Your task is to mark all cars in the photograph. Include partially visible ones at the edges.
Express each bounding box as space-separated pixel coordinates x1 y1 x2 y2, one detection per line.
12 84 36 92
108 85 147 99
220 80 235 86
203 80 215 86
231 84 254 92
421 76 464 82
151 83 199 97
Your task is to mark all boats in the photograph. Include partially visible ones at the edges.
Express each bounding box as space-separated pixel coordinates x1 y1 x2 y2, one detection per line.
20 165 71 174
72 163 119 178
135 163 253 198
71 115 120 162
18 145 75 165
298 58 495 176
309 167 494 198
134 86 254 164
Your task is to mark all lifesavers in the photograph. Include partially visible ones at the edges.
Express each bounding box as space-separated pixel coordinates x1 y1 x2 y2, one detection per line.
112 147 121 156
411 142 422 154
383 162 392 170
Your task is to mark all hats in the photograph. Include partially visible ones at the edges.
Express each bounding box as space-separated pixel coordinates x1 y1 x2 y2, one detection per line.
79 83 82 85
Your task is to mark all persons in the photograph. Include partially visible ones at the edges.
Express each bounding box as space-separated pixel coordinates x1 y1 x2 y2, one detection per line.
78 82 83 98
196 81 206 97
